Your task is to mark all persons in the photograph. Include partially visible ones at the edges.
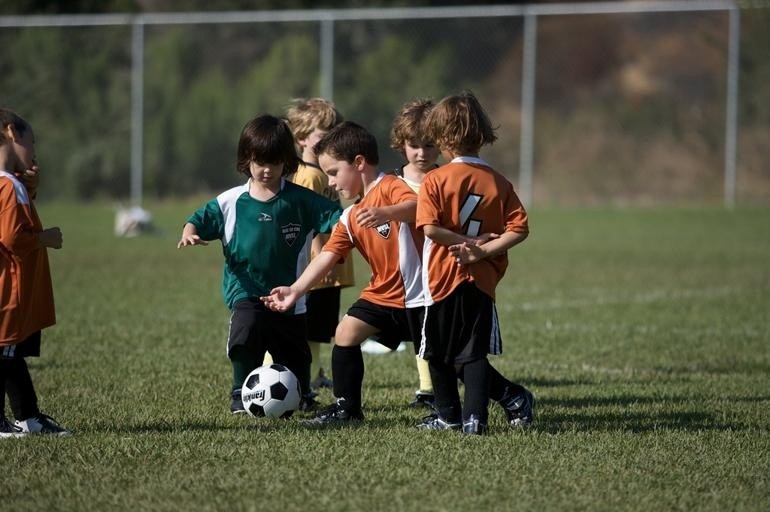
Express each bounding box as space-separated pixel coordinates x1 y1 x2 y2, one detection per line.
178 114 347 414
416 90 531 436
1 107 68 439
284 96 355 392
383 95 440 408
260 121 534 432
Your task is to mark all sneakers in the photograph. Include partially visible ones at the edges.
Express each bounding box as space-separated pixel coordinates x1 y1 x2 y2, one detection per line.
14 414 68 435
0 417 26 438
311 367 333 390
409 390 436 409
303 403 365 428
413 413 463 430
229 388 246 413
460 415 489 435
502 383 535 428
299 392 320 415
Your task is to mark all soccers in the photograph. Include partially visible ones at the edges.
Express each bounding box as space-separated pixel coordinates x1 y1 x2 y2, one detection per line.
241 364 301 418
